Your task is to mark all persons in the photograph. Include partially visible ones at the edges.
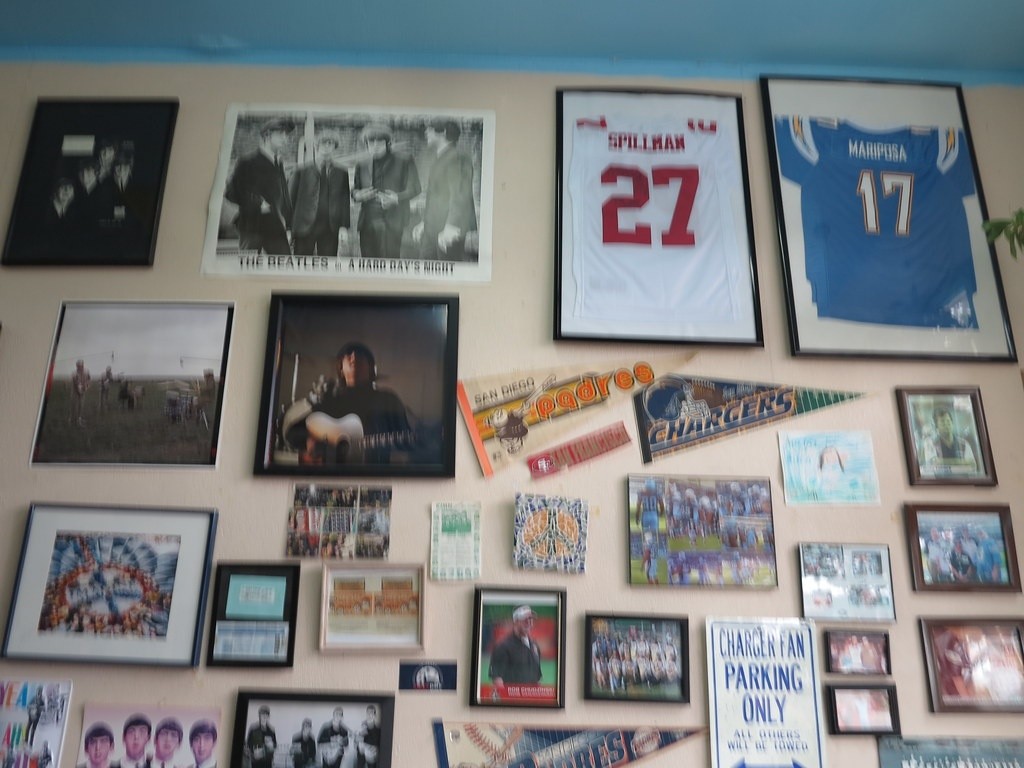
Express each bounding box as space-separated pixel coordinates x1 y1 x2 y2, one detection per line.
247 705 277 768
924 409 976 479
117 378 136 411
77 712 217 768
289 717 317 768
590 631 679 693
288 486 389 559
222 117 478 264
99 366 113 408
926 525 1003 583
43 138 142 261
832 633 887 672
488 605 546 694
37 536 171 640
317 704 381 768
24 686 44 746
66 360 92 431
277 342 414 466
636 482 774 586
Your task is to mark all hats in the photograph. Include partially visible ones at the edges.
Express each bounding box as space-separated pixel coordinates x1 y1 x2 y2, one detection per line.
114 149 134 168
53 176 75 193
258 116 295 136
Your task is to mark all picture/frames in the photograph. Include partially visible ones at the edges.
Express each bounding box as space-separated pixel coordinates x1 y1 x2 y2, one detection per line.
2 501 219 670
903 501 1023 593
229 688 396 768
919 613 1024 715
895 383 998 487
319 561 425 653
206 559 300 668
584 611 691 703
1 93 180 270
824 628 892 676
468 584 566 710
824 681 901 737
758 73 1017 365
798 541 897 624
553 86 765 347
254 293 461 477
